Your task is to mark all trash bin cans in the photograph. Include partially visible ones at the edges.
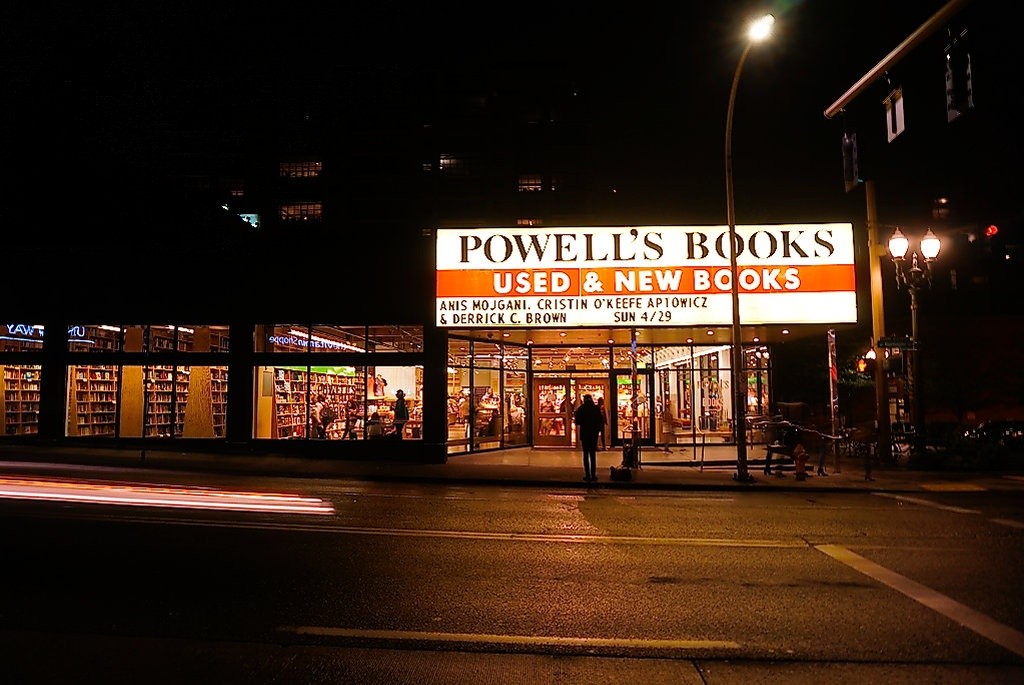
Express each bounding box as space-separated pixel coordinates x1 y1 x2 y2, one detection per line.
623 446 638 467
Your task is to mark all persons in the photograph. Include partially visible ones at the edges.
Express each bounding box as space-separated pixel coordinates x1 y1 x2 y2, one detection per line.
784 402 830 480
366 413 384 438
595 397 608 451
376 374 388 396
455 387 526 424
574 394 604 481
560 393 575 434
473 409 500 448
340 400 359 441
390 390 408 433
312 395 330 438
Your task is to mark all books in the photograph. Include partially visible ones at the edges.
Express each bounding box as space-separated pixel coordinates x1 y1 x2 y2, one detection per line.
273 369 366 436
142 333 190 438
4 363 41 435
72 325 120 437
211 334 229 438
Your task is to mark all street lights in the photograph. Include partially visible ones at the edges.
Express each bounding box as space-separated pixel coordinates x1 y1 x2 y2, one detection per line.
888 225 943 469
723 13 776 481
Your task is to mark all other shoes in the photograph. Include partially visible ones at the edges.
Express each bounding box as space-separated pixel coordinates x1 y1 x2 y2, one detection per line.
583 476 592 482
591 476 598 481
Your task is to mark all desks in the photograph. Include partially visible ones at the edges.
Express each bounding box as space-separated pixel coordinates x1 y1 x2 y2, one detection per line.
745 416 790 451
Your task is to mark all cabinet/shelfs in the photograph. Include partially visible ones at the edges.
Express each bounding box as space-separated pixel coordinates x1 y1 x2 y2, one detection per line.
118 325 193 438
0 338 42 434
68 325 126 436
181 324 230 439
254 328 375 439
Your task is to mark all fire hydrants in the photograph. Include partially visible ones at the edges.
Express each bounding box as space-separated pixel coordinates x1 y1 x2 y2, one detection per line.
793 444 810 480
